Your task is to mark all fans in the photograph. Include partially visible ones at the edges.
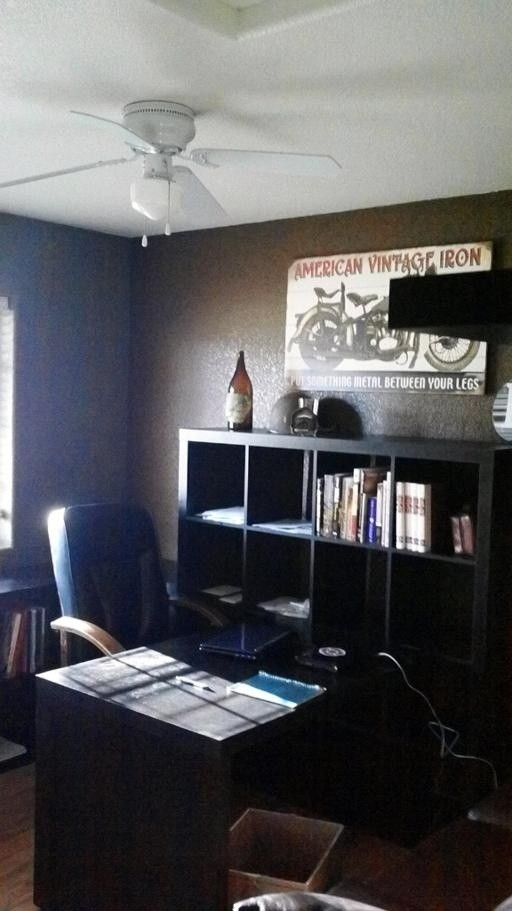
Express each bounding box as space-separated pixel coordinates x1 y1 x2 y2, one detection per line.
0 100 342 216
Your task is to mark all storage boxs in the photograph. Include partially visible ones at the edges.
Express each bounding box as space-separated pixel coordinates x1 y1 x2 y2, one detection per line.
228 808 344 911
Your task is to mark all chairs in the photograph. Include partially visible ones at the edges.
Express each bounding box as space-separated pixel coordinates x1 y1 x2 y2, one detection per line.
47 503 229 666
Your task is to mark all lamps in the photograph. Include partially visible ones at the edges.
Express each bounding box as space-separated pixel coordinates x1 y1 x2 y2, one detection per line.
129 158 182 248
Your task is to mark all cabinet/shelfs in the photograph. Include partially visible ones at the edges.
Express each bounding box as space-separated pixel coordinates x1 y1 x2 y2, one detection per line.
0 572 62 771
175 428 512 812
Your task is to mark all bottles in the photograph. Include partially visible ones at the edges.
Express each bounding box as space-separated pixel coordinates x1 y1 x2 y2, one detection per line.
225 351 253 432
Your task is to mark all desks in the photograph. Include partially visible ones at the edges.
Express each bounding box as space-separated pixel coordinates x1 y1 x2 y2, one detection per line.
35 629 415 910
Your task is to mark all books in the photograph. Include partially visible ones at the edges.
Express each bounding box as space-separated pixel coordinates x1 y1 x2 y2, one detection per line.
229 670 327 708
251 518 312 536
1 603 55 679
315 467 475 559
201 507 244 525
